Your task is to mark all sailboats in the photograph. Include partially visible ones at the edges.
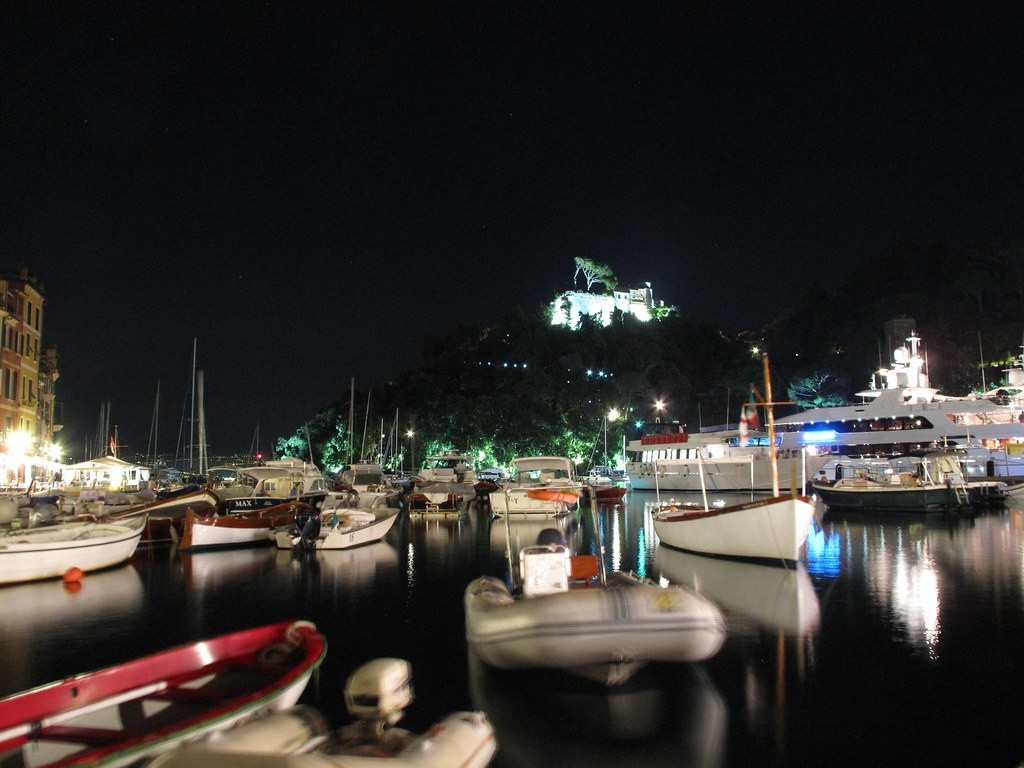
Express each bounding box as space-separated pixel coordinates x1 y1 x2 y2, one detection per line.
651 351 819 562
649 535 821 763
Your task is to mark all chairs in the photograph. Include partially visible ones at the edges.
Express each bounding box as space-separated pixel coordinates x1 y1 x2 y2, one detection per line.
518 544 573 598
569 555 599 587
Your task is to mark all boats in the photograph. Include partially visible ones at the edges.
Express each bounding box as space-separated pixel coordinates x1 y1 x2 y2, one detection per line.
3 336 630 548
809 433 1010 517
178 501 306 555
181 542 279 592
462 540 728 693
0 508 149 585
621 387 838 495
148 653 497 768
756 329 1004 481
1 616 330 768
276 505 401 550
